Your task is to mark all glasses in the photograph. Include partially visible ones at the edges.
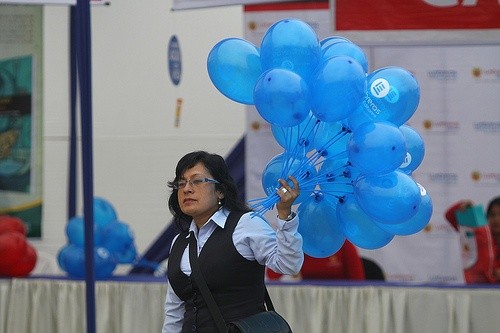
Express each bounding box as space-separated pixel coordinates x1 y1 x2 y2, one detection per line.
175 176 219 190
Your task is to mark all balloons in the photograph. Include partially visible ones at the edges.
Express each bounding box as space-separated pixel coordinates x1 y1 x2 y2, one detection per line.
58 197 139 279
207 19 433 258
0 215 37 278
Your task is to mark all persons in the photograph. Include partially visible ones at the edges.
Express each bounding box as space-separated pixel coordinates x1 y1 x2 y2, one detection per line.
445 197 500 286
265 240 364 282
162 151 305 333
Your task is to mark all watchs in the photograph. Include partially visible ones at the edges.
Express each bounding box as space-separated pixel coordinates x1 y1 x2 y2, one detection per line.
280 211 295 221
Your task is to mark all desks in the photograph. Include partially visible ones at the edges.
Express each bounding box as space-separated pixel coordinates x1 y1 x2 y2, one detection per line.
0 274 500 333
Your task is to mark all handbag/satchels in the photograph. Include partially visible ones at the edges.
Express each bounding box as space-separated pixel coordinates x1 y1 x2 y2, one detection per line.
227 311 293 333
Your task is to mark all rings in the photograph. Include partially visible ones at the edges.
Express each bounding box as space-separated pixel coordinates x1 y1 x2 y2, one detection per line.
281 188 287 194
